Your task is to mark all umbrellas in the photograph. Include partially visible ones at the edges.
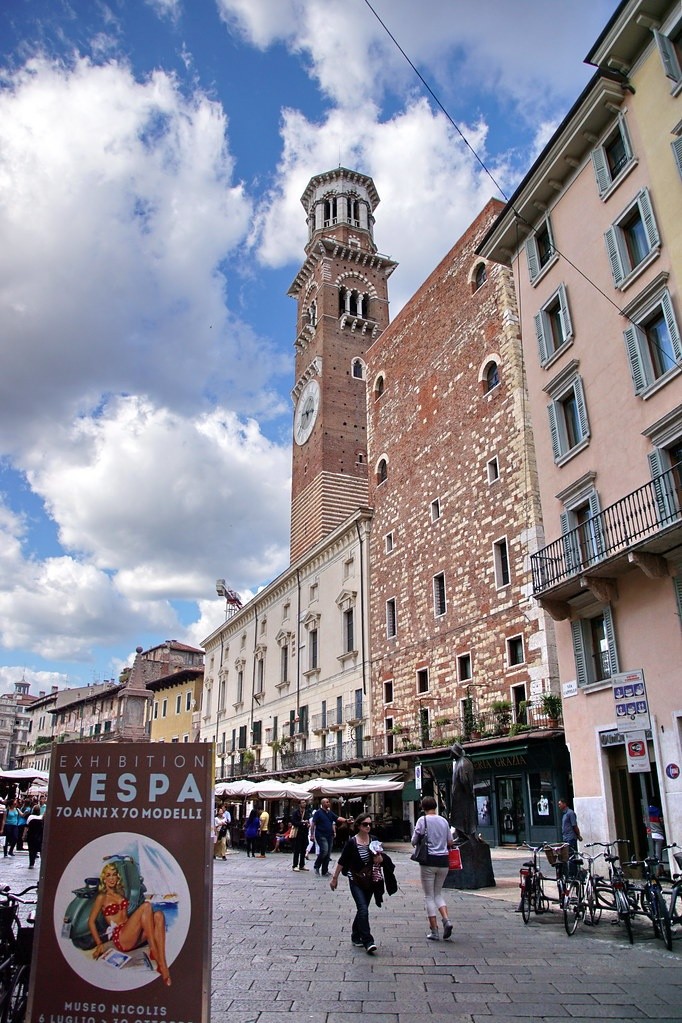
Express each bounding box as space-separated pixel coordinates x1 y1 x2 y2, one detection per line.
215 777 404 828
0 768 49 802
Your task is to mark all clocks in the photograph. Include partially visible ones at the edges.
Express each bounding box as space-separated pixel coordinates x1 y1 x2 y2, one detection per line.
294 379 321 446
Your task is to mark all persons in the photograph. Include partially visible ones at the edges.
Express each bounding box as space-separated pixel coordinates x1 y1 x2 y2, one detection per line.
558 799 583 879
0 795 47 869
450 742 478 842
411 796 453 940
643 796 664 878
213 798 354 877
330 812 383 952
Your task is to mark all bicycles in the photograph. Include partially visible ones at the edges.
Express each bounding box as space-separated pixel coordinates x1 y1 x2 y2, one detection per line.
0 882 38 1023
544 841 570 911
562 851 606 935
516 841 552 923
624 855 673 952
585 838 639 945
663 843 682 925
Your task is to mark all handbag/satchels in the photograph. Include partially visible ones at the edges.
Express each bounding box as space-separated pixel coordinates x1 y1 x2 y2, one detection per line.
289 825 298 839
410 816 428 863
449 846 463 870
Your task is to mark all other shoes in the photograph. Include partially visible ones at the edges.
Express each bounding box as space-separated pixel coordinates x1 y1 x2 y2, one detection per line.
314 867 320 876
35 855 39 858
366 940 377 952
255 854 265 858
427 926 439 941
442 917 454 939
222 856 227 860
305 856 309 860
271 848 281 853
293 867 300 872
8 850 15 856
323 872 333 877
351 933 364 947
299 867 309 871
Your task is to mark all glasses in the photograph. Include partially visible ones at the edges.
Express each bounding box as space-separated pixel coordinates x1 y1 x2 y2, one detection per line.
360 822 373 827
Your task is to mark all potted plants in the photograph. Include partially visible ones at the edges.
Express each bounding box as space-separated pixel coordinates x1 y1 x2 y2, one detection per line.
518 700 534 716
415 714 433 747
539 694 564 727
486 700 515 713
217 733 303 758
435 718 450 726
497 714 512 734
459 712 486 740
243 749 254 770
393 723 410 734
386 729 394 735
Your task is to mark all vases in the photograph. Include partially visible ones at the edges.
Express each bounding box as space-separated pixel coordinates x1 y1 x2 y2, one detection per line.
313 729 328 735
399 746 407 751
329 724 342 731
364 735 372 741
254 765 262 774
347 720 360 726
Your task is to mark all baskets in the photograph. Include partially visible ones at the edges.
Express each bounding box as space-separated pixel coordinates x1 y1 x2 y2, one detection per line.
544 843 570 864
674 852 682 870
622 861 646 880
